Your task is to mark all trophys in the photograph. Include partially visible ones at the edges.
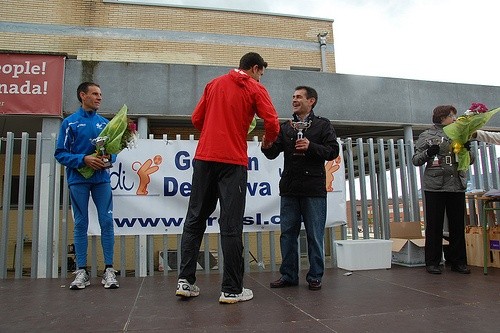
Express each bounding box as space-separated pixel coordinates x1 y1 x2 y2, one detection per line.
89 136 113 169
290 119 312 143
425 137 446 169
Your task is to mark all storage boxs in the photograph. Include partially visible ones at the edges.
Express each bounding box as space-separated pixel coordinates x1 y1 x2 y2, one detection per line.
158 249 219 271
331 239 394 271
389 221 449 266
464 225 500 267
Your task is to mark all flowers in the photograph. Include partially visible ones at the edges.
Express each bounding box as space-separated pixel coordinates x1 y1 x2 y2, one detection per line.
456 102 488 121
119 118 136 151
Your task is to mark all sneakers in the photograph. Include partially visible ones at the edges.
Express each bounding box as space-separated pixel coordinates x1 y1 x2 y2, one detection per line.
101 268 120 288
219 286 254 303
175 280 201 297
70 269 90 290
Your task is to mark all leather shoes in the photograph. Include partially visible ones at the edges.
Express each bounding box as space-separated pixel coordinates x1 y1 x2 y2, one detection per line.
452 263 471 274
427 264 443 274
310 283 322 290
270 275 300 287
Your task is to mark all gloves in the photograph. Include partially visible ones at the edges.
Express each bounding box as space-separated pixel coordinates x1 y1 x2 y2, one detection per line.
428 144 440 156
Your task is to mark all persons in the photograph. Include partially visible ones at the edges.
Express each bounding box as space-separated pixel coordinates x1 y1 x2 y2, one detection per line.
261 86 339 291
470 129 500 145
412 105 472 274
54 82 120 290
176 52 280 303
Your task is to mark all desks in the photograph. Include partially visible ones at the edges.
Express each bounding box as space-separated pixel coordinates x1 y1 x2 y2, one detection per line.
475 197 500 275
